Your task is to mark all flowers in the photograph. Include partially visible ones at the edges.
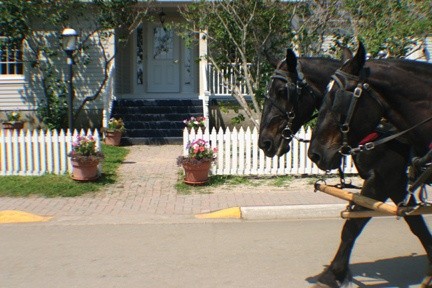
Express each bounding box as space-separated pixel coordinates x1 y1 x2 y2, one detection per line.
186 137 218 163
182 115 208 129
67 135 105 168
107 117 129 134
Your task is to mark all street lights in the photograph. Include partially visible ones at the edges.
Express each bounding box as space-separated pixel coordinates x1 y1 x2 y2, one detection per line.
60 25 78 135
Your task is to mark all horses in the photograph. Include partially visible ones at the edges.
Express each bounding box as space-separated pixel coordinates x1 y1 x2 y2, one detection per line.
257 46 432 288
304 41 432 174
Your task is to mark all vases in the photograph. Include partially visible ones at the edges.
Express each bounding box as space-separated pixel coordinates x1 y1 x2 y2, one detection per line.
66 151 100 182
105 131 122 147
188 128 206 134
182 159 212 185
